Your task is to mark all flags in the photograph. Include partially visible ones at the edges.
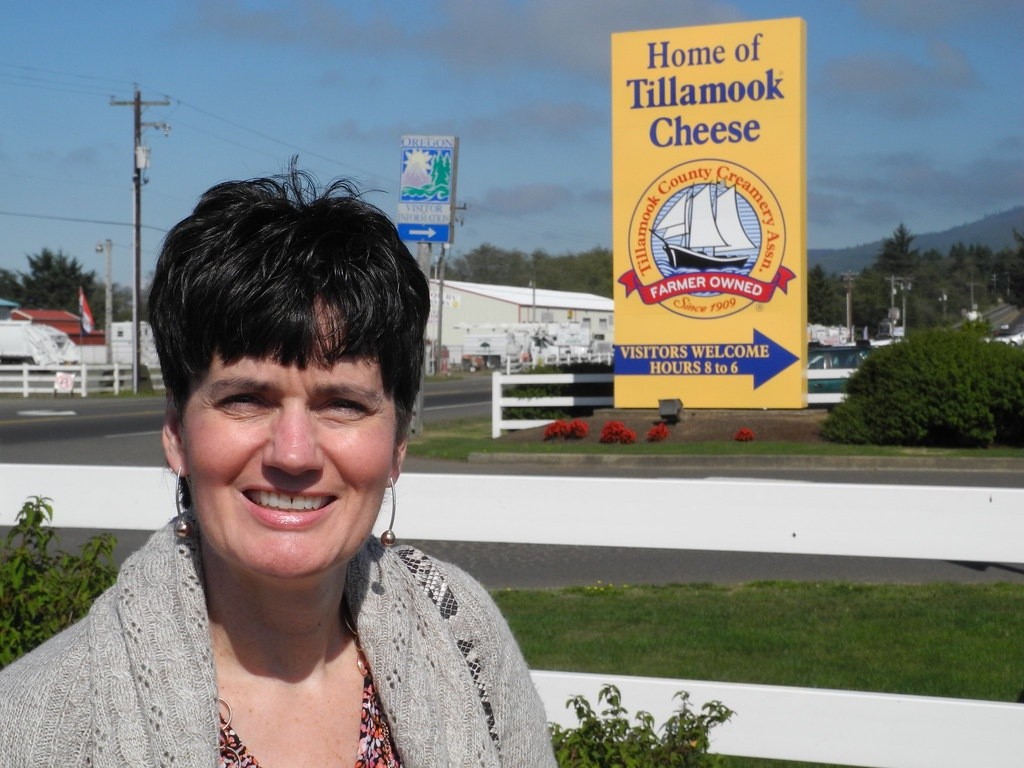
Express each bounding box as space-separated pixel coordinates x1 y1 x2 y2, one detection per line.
79 286 94 335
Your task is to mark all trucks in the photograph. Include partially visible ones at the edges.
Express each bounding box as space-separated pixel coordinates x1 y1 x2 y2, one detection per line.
0 319 81 374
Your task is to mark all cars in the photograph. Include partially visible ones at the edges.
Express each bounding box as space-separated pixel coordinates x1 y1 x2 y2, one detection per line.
806 344 876 395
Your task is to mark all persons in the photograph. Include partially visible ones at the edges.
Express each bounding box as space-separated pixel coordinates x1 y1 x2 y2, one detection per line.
0 170 557 768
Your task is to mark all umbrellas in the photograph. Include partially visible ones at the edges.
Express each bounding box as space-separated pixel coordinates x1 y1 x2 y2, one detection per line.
452 321 478 334
